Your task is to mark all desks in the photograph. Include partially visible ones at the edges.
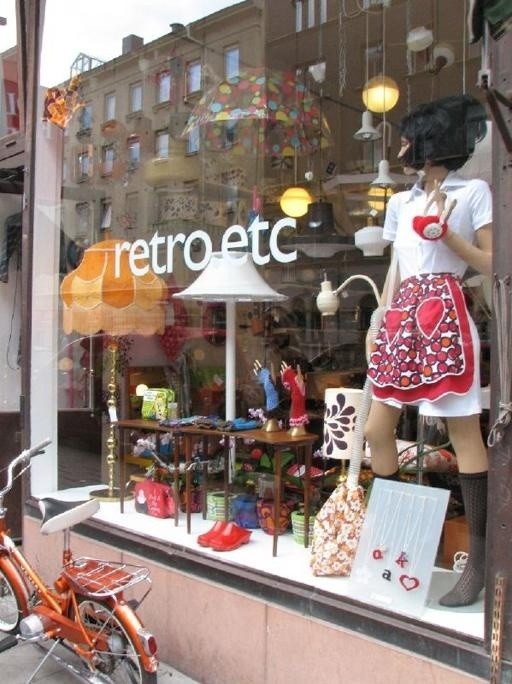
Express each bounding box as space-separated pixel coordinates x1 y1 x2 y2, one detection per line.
106 417 319 557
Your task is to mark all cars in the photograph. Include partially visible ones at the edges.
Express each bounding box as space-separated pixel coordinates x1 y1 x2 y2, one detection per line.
306 340 367 409
59 327 250 454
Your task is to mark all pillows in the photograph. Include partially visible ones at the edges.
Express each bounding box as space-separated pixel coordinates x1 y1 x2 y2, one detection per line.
314 437 459 475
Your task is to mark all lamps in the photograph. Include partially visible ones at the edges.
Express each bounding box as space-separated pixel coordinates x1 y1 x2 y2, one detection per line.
172 251 290 485
315 268 382 318
279 3 459 257
322 388 366 488
59 237 170 502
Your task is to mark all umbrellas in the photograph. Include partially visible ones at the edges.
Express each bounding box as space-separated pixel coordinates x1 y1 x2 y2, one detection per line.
179 66 336 186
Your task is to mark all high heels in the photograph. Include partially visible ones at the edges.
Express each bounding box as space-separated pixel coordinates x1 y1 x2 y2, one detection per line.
197 520 253 551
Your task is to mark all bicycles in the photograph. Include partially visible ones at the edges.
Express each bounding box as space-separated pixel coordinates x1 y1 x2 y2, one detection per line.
0 438 157 684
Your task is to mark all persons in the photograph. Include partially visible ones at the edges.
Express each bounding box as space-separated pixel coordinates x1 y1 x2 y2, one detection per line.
363 94 494 607
251 358 317 427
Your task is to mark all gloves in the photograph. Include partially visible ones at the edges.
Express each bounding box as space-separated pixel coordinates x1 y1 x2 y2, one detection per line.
279 365 310 426
254 367 283 420
159 413 264 432
